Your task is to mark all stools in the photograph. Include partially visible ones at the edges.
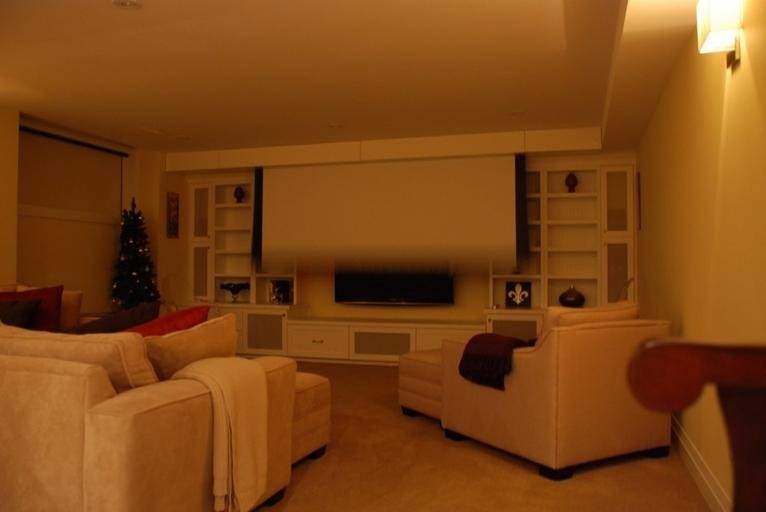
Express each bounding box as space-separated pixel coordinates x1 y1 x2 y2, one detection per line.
292 370 332 468
398 346 442 423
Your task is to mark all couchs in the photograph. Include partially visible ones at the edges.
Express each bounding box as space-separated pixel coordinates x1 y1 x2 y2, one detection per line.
0 355 296 512
439 318 681 480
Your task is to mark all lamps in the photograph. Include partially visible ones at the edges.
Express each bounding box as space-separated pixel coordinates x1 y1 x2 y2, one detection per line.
696 0 741 69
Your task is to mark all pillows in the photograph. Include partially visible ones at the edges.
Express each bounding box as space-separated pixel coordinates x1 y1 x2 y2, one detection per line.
1 323 159 394
0 282 64 336
541 299 640 339
0 298 40 329
75 300 159 331
120 304 211 338
16 285 83 333
144 312 237 381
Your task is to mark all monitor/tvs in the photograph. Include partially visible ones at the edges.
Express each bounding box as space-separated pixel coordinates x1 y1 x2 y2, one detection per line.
334 270 455 307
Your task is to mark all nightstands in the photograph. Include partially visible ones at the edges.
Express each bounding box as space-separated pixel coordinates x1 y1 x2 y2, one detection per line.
253 274 295 309
542 170 602 309
491 168 543 312
211 179 254 309
601 166 638 312
186 182 211 303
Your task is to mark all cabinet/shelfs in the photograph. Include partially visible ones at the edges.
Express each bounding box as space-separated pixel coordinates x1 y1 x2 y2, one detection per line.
212 304 549 376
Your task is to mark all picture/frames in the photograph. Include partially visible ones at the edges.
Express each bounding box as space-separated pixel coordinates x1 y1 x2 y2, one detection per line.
166 191 181 241
